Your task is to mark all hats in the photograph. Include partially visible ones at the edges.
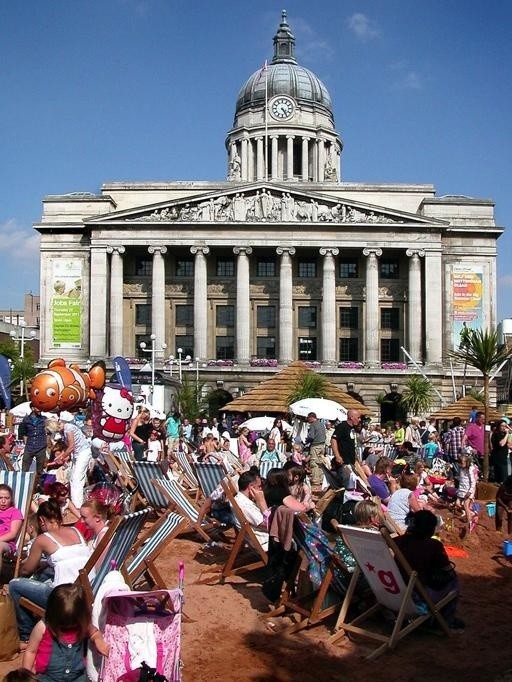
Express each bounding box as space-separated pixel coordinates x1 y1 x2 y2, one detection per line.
222 430 230 440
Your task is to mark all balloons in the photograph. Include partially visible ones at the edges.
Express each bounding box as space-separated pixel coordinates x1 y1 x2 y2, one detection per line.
29 358 138 443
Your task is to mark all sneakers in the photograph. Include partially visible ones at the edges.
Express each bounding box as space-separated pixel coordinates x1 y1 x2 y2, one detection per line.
19 641 28 652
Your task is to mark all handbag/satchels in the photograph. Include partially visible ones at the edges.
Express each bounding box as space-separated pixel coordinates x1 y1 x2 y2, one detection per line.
429 561 456 590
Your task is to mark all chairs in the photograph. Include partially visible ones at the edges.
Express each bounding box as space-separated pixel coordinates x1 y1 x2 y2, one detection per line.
0 451 459 665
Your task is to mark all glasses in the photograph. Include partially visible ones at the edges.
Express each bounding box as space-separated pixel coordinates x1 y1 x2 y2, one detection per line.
60 491 68 496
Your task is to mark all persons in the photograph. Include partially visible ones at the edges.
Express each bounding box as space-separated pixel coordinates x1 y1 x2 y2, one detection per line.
22 583 108 681
0 397 512 651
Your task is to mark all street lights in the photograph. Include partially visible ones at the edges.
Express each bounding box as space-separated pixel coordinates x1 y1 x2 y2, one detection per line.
8 322 36 396
139 334 207 402
